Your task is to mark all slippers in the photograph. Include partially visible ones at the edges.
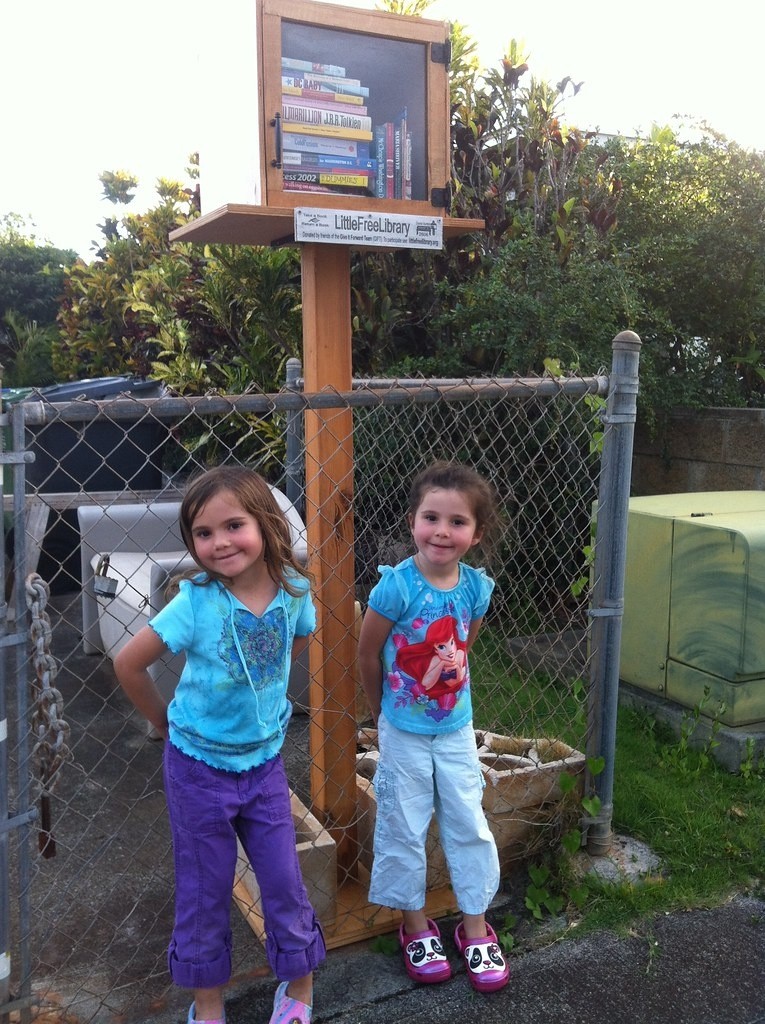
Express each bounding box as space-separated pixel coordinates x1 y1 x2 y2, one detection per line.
269 981 313 1024
188 999 226 1024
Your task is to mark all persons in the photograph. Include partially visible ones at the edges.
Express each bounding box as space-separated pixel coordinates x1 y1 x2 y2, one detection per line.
357 463 510 992
114 467 326 1024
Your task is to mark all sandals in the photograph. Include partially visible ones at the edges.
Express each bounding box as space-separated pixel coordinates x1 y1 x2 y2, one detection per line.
454 920 510 993
399 916 451 982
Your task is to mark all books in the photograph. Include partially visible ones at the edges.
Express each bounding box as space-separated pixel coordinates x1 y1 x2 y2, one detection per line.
279 56 413 203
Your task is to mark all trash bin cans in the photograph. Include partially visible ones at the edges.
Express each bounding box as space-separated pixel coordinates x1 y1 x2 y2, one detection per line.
1 388 53 597
24 376 165 596
